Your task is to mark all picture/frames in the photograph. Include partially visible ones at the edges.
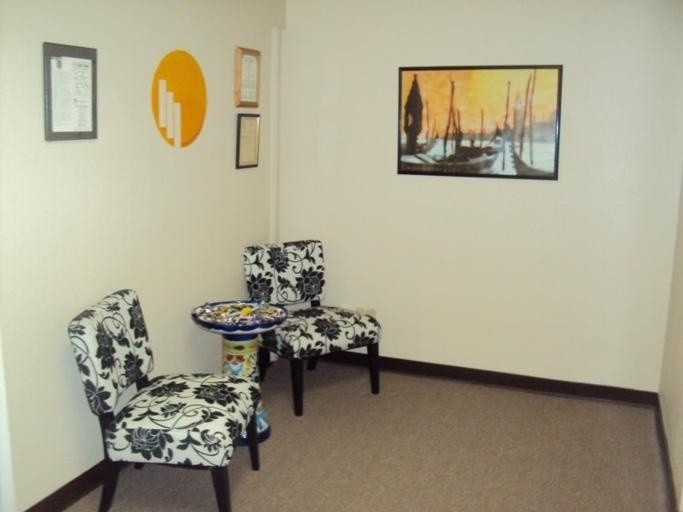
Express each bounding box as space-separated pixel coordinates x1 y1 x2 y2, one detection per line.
236 113 260 169
397 64 563 181
234 46 261 108
43 42 98 142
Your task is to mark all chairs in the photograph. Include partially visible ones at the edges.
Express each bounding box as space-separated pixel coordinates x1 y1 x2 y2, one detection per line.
242 240 383 416
66 288 263 512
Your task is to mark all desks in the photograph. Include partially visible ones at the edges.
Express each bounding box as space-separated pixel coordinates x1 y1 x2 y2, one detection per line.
191 299 288 447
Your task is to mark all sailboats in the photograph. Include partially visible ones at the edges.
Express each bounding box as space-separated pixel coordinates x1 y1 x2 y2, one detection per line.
396 69 553 175
